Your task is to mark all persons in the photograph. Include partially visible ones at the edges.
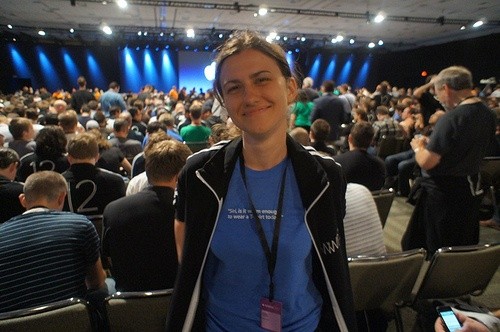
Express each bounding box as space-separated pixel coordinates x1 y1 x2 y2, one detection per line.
0 66 500 332
173 30 356 332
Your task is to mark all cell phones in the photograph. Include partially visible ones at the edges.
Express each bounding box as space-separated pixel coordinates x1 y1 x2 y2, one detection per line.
436 305 462 332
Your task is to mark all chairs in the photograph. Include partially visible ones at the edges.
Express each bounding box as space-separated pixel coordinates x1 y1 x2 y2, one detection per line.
474 156 500 222
377 134 407 159
0 187 500 332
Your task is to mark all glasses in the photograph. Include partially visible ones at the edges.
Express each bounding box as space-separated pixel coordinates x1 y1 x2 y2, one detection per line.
434 93 443 101
